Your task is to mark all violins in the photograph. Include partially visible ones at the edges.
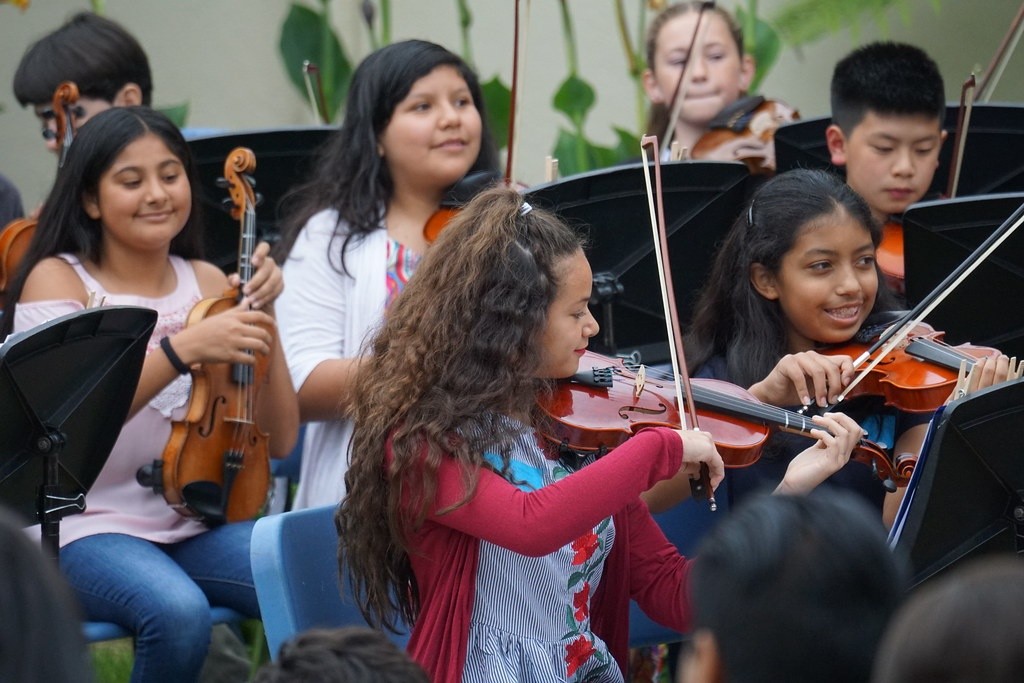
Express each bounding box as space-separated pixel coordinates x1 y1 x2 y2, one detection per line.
0 81 79 310
534 348 923 495
689 100 798 175
160 147 277 525
876 216 910 293
811 315 1001 412
422 179 534 253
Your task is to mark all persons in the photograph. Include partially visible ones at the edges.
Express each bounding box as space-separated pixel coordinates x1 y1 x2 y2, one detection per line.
333 186 864 683
625 168 1023 535
1 104 299 682
12 12 154 155
825 40 949 233
643 1 755 165
1 507 1024 683
272 37 501 517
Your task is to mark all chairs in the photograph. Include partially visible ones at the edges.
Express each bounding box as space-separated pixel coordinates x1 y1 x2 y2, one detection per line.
249 503 417 668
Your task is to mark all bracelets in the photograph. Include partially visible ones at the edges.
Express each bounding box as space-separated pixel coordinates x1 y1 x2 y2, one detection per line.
160 337 190 376
